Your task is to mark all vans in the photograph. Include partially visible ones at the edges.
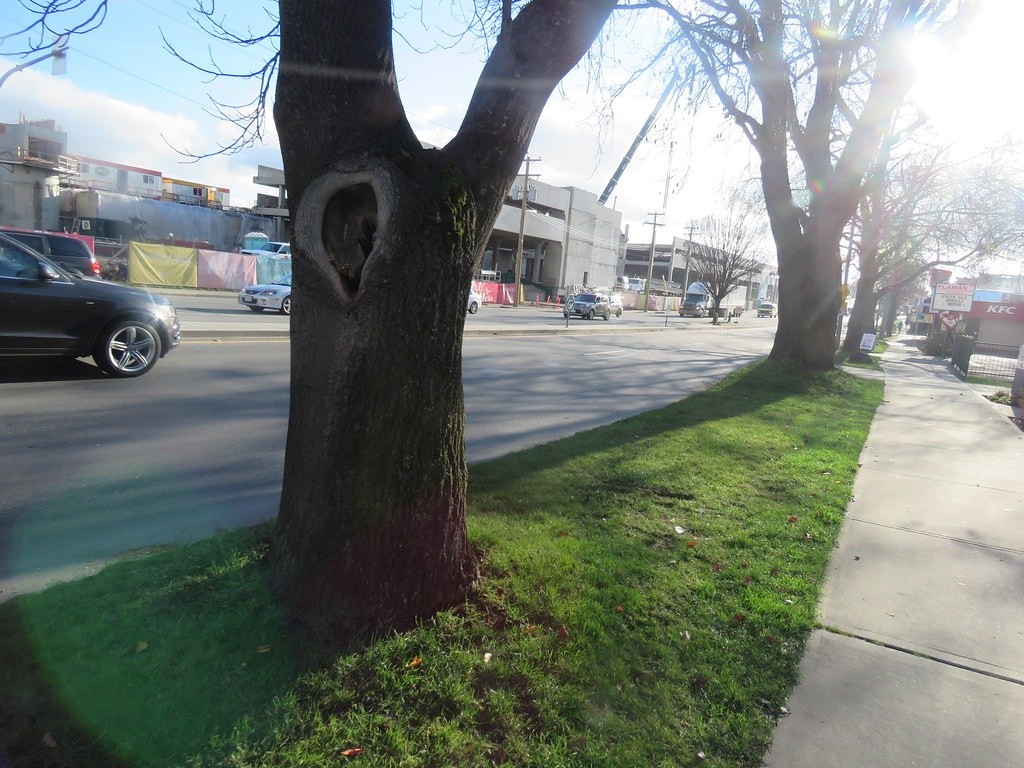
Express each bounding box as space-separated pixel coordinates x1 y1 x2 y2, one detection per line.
0 225 94 255
757 303 778 318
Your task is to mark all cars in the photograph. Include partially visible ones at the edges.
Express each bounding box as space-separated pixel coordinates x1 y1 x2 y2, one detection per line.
843 309 849 316
562 292 611 321
594 290 623 318
467 289 481 314
237 273 292 316
0 234 181 379
241 242 290 259
874 314 880 319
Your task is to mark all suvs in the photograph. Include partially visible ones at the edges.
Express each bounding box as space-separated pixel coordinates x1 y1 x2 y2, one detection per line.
0 233 100 280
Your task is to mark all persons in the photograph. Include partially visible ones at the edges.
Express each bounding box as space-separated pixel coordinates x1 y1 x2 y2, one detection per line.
898 321 903 334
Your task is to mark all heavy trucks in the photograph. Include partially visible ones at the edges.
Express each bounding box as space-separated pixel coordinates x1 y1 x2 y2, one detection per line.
678 281 747 317
616 277 646 294
